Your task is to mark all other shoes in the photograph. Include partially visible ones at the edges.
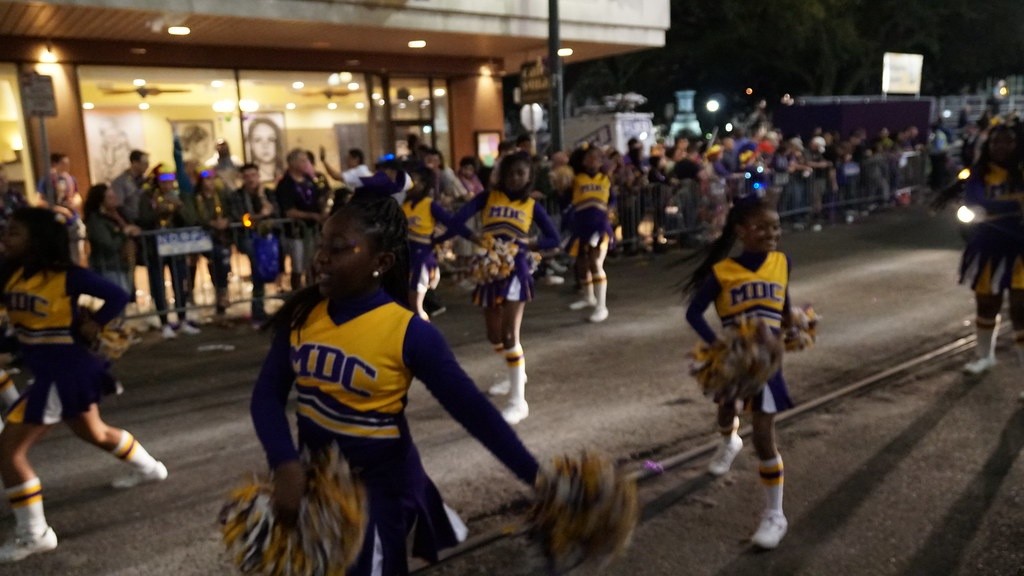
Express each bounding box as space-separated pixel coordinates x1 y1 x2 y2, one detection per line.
589 307 608 322
180 322 201 336
504 400 529 425
161 323 176 340
569 298 597 310
961 357 995 375
488 373 527 396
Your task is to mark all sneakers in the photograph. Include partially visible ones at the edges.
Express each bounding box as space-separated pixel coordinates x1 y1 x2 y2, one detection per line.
751 513 788 548
708 435 743 475
112 461 168 488
0 526 59 564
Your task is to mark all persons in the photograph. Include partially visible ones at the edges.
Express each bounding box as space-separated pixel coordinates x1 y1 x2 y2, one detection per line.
383 125 947 276
249 189 546 576
684 198 798 548
50 148 374 343
451 152 560 425
248 117 284 180
566 144 618 323
0 208 169 562
959 98 1024 369
399 167 477 322
181 126 211 166
